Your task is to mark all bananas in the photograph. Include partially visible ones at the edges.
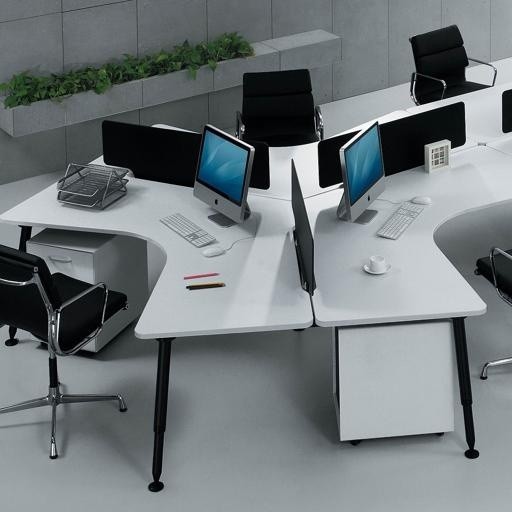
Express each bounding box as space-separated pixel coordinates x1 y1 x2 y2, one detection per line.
375 200 423 241
159 212 216 248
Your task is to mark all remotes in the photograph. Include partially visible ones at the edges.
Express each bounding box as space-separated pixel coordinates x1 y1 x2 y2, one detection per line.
202 246 226 257
411 196 431 204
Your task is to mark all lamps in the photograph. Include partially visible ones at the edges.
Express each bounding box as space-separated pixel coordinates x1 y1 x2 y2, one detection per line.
474 246 512 380
234 68 324 148
0 244 129 460
408 24 497 106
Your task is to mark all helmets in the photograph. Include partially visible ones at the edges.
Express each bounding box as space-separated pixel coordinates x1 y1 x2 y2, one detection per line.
364 264 391 275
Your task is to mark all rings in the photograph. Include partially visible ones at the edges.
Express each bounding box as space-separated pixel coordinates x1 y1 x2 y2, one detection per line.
186 283 225 290
184 273 219 280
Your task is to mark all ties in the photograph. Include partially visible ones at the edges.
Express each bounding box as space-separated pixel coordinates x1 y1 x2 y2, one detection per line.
192 123 255 230
336 120 387 226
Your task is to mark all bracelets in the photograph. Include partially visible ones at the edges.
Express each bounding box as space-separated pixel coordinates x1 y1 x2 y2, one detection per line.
369 256 384 271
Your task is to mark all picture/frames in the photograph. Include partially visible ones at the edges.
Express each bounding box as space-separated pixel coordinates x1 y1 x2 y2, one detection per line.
404 79 512 144
485 133 512 156
303 145 512 459
0 169 315 492
81 108 479 201
